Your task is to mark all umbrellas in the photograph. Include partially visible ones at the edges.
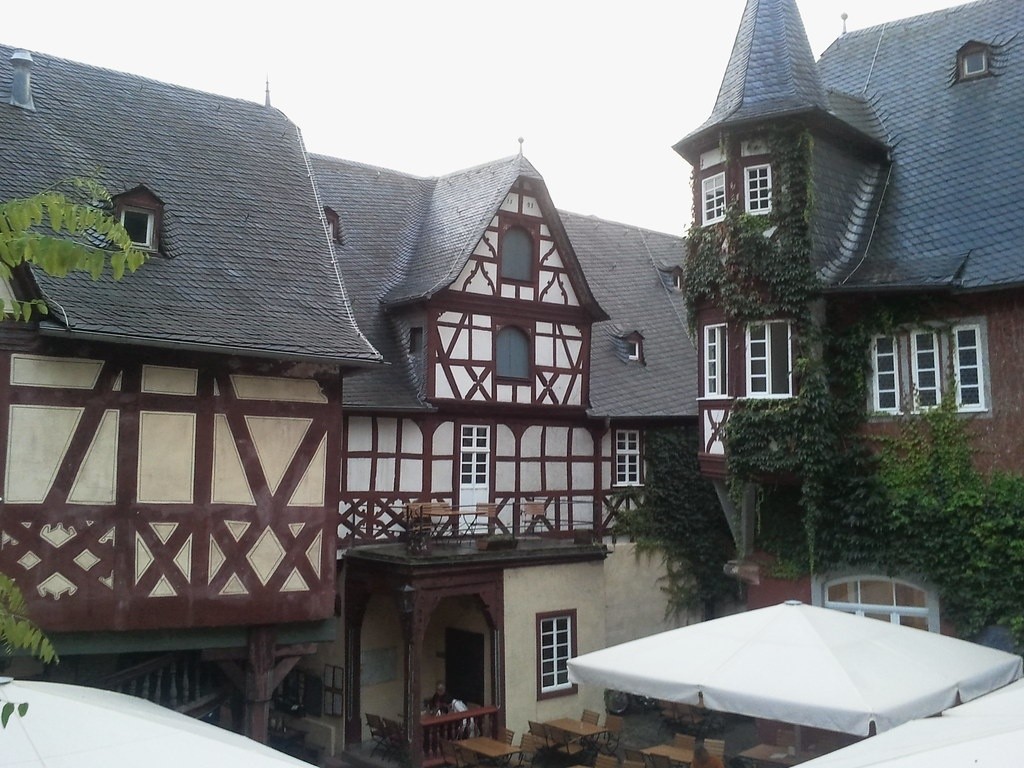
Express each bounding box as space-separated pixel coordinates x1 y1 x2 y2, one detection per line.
0 676 319 768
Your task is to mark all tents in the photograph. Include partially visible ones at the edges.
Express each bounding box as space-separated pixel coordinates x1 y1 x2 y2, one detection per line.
566 598 1023 746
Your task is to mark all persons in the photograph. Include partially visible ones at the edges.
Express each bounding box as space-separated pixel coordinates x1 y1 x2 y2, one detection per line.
439 694 481 739
432 681 448 715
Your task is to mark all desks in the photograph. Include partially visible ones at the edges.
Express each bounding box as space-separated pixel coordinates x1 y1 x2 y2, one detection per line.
457 737 523 768
421 509 486 548
544 719 609 760
640 744 694 768
739 743 820 768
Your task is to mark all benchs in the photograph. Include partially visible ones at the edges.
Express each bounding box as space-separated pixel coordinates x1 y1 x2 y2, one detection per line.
402 502 459 549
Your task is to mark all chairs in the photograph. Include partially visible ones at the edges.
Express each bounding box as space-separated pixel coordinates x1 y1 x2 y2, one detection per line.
470 503 497 545
365 712 408 765
522 503 546 542
623 730 795 768
439 708 625 768
406 504 436 552
